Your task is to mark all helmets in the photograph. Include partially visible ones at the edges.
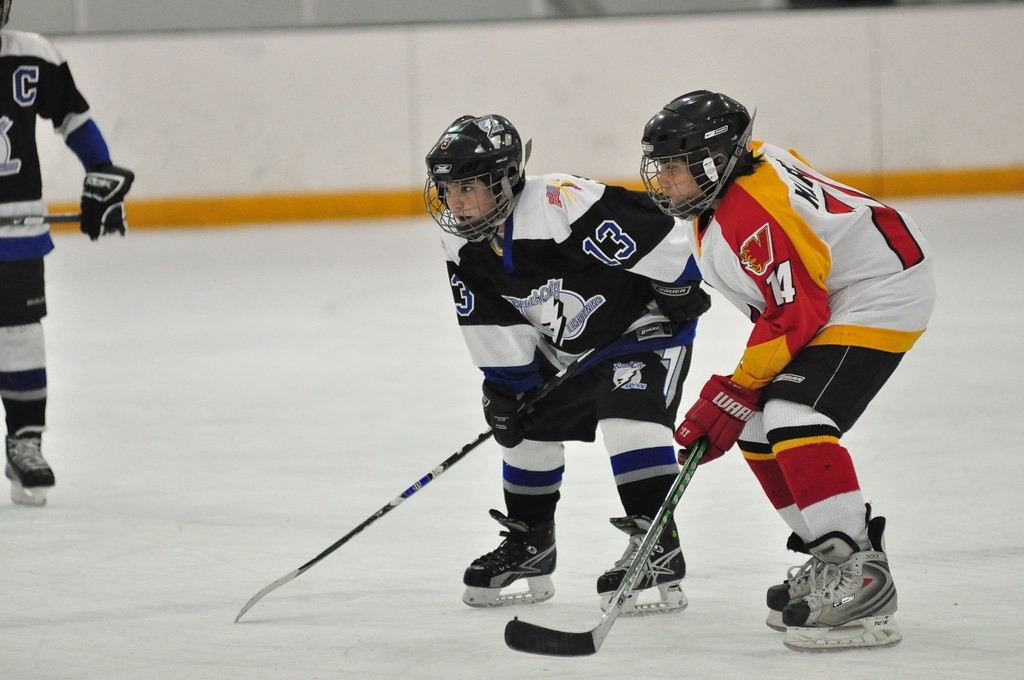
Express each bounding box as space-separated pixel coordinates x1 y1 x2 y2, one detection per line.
425 115 523 198
641 92 751 200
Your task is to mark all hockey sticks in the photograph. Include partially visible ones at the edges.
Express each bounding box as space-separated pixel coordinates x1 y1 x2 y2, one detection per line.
0 210 80 226
235 343 608 626
502 435 715 660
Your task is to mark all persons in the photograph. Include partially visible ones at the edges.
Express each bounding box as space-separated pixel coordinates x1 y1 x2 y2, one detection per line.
0 1 133 507
640 91 937 653
423 115 711 616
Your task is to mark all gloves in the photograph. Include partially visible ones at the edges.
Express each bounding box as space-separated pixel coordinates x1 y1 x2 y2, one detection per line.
481 378 543 448
650 278 712 323
675 375 762 465
80 165 135 242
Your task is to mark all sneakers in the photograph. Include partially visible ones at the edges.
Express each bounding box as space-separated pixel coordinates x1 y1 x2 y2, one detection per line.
462 509 558 608
5 435 55 505
766 532 820 633
596 512 689 618
782 515 904 652
